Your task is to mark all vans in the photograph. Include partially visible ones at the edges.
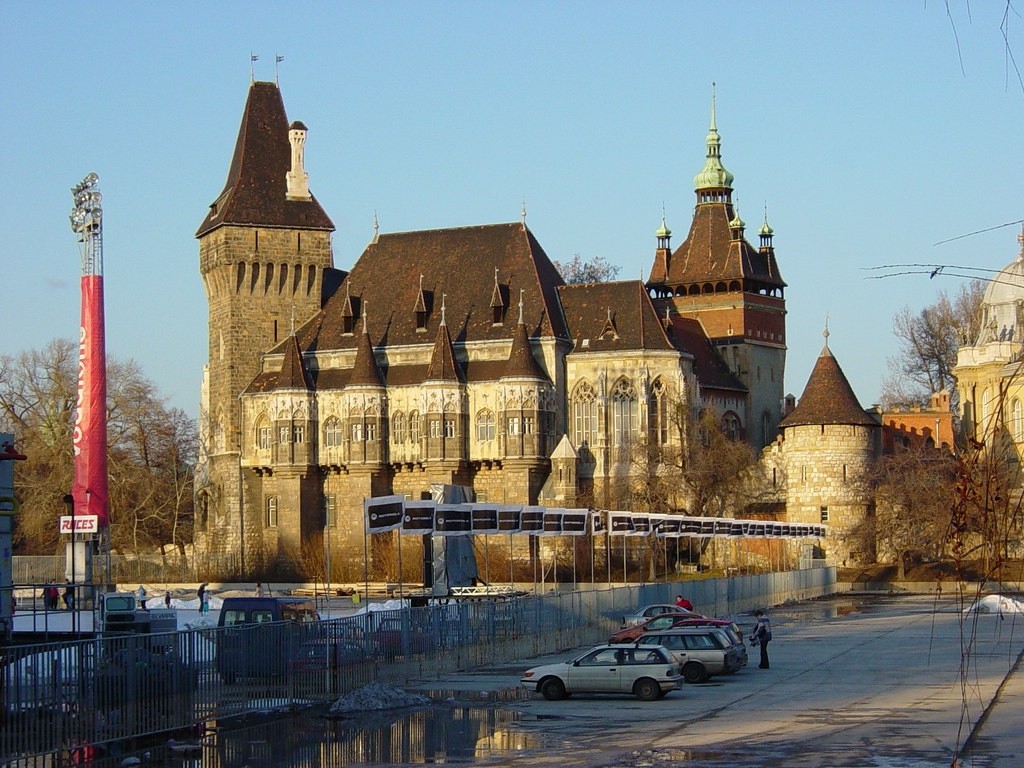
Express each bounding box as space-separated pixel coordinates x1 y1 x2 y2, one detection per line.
216 596 332 685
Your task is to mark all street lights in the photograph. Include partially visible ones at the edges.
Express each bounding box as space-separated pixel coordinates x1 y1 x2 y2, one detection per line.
63 493 76 635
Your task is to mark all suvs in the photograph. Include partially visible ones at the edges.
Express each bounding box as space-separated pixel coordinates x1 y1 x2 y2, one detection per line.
596 629 740 683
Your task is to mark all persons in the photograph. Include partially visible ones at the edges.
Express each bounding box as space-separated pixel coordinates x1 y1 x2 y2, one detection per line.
51 588 60 611
203 590 212 613
255 581 264 598
749 611 772 670
165 591 170 610
63 578 73 609
138 585 147 610
40 588 51 611
675 595 694 613
196 581 209 612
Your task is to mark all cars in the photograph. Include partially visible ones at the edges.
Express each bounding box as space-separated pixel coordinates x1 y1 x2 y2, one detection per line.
608 613 707 643
318 623 381 653
519 641 685 700
290 639 376 686
366 617 434 654
665 621 748 671
620 605 714 627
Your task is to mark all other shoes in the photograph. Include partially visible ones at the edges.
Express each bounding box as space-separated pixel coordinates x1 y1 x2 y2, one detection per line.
759 664 769 669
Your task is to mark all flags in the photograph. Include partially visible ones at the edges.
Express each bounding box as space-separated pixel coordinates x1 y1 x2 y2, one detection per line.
364 494 406 535
461 502 499 535
520 505 546 536
564 507 588 536
434 502 473 537
592 509 827 540
402 498 436 536
543 507 565 537
498 504 525 534
